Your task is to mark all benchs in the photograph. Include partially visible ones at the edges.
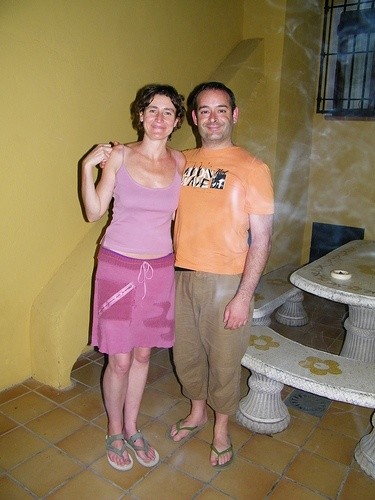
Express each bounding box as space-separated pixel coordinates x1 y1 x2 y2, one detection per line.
253 263 309 327
235 325 375 480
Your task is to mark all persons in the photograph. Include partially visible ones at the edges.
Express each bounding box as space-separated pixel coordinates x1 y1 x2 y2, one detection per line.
82 84 184 471
173 81 275 466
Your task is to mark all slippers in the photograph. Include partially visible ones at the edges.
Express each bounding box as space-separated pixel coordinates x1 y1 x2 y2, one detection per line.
122 433 159 467
106 434 133 471
169 416 210 444
210 434 233 468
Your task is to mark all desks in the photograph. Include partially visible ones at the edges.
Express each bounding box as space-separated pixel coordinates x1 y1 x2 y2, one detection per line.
290 240 375 361
